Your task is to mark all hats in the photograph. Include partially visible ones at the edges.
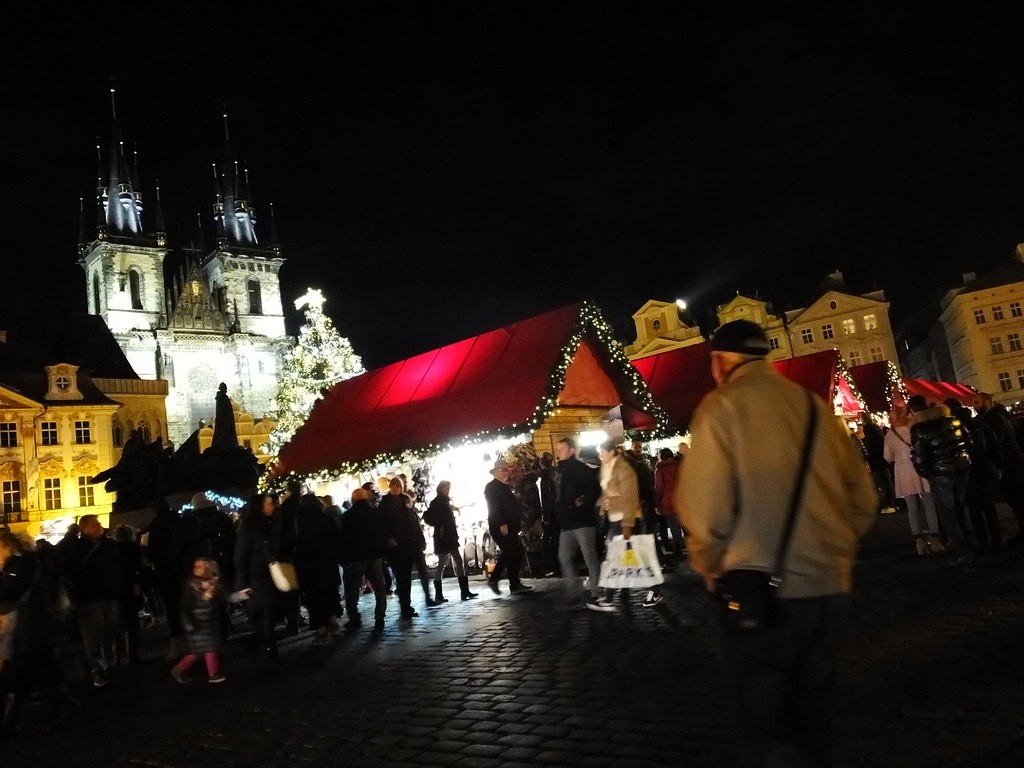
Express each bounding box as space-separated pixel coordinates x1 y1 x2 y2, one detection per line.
191 493 217 512
709 320 770 355
111 524 133 542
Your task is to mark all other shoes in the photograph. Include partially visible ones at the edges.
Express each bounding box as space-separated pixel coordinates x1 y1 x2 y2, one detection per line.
510 583 532 594
586 597 616 612
114 654 136 665
916 534 945 555
643 591 664 607
400 607 415 616
289 623 301 635
344 618 361 630
311 633 330 644
375 619 385 628
487 580 501 595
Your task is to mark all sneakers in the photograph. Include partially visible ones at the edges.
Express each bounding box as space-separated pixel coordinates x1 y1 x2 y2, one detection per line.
171 668 190 684
93 675 110 686
209 675 225 684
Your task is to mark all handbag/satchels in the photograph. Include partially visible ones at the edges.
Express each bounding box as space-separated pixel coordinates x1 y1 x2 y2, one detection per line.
712 568 780 636
598 533 665 589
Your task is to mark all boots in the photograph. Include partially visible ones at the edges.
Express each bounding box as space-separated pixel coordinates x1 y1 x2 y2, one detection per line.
458 576 478 600
420 580 437 606
434 580 448 603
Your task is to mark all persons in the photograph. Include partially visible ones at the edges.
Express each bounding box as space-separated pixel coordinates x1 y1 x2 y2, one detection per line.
484 392 1024 611
212 382 238 446
672 320 877 768
0 473 479 729
